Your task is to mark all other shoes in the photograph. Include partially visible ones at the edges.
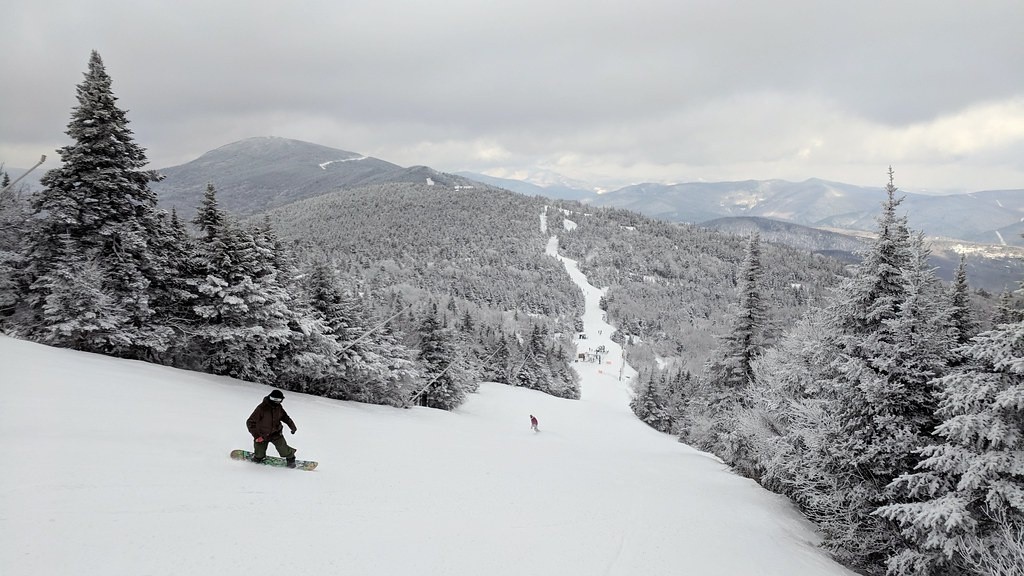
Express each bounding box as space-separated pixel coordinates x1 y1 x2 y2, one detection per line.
252 456 261 463
287 458 296 469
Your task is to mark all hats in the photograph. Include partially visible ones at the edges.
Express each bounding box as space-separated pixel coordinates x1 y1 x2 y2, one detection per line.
270 390 284 402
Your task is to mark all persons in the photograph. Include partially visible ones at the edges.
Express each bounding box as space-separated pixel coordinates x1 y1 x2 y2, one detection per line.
530 415 540 432
246 390 297 463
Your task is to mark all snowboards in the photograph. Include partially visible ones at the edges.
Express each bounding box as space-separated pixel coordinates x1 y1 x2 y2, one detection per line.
230 449 318 471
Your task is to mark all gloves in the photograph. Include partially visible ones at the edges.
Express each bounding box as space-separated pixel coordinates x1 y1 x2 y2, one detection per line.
255 437 264 443
291 427 296 434
